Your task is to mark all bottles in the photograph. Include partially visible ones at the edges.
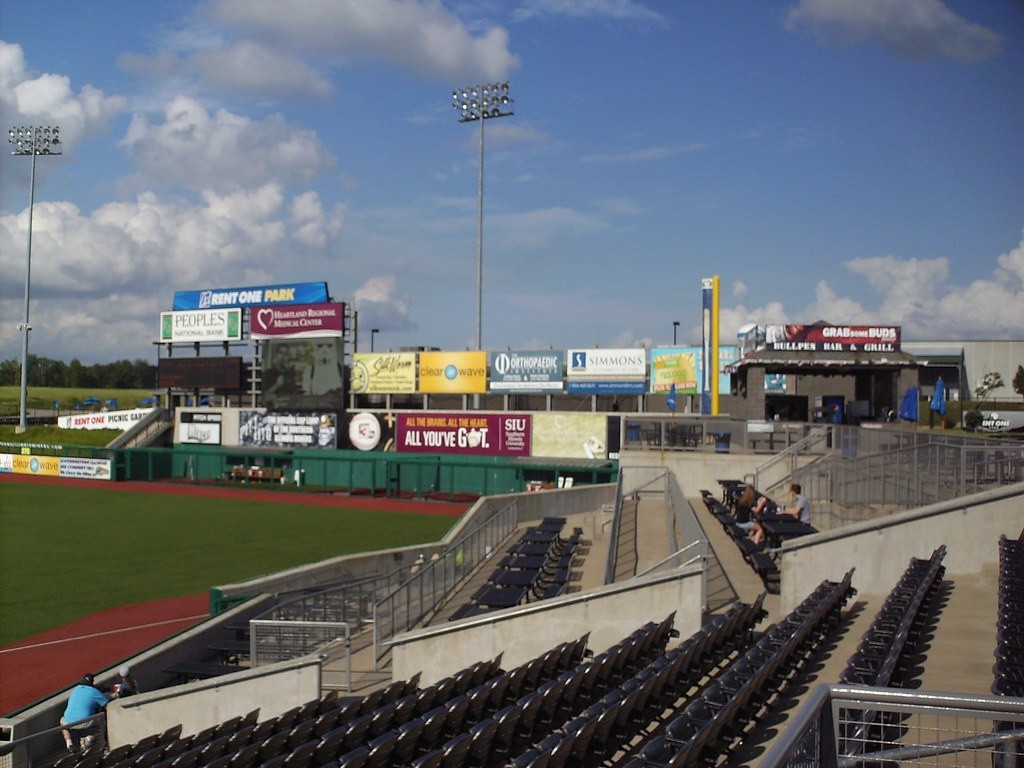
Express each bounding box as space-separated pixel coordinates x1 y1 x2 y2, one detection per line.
781 503 785 512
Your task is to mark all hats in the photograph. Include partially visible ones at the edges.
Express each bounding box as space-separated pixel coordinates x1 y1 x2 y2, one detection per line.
119 666 130 676
84 673 94 682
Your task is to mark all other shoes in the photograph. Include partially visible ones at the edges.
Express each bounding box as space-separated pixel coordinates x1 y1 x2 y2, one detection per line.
66 739 73 753
85 735 93 747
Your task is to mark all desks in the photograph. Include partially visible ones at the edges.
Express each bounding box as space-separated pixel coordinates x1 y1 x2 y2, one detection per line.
650 421 704 447
718 480 819 562
462 517 567 619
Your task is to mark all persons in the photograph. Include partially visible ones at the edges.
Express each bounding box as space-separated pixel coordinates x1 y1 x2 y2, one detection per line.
776 484 811 527
736 485 766 545
117 666 139 698
60 673 110 752
262 343 298 394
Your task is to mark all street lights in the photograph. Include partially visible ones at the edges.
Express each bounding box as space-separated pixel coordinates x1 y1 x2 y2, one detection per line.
450 81 515 349
671 320 681 344
370 329 379 355
8 124 68 427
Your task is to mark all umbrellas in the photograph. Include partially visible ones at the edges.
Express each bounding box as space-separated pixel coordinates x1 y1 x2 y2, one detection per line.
187 399 193 406
929 376 946 432
667 382 676 428
900 385 920 447
199 397 215 405
55 397 160 411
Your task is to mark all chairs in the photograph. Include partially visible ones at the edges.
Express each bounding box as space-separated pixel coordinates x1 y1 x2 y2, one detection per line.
51 527 1024 768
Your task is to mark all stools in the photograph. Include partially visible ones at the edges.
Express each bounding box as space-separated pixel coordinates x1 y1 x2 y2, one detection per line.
639 430 718 447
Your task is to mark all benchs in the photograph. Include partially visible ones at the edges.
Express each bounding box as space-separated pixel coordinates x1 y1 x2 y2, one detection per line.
227 469 282 482
448 526 582 623
701 490 780 594
